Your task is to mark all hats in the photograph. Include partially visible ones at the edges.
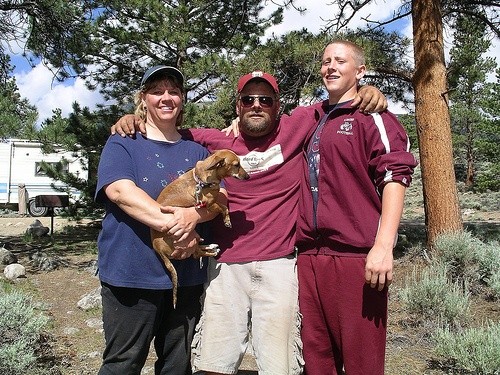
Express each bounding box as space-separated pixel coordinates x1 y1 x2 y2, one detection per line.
140 64 186 88
236 70 279 95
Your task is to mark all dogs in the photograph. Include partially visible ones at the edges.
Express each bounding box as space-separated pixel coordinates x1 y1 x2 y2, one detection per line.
150 149 251 309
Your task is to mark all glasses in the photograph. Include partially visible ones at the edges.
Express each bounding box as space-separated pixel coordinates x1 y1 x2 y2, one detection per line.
238 95 278 108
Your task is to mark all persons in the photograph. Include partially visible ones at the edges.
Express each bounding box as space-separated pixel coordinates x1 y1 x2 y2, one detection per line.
95 65 229 375
221 41 419 375
110 70 388 375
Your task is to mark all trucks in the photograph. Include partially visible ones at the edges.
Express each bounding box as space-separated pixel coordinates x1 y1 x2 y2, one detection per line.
0 137 91 217
